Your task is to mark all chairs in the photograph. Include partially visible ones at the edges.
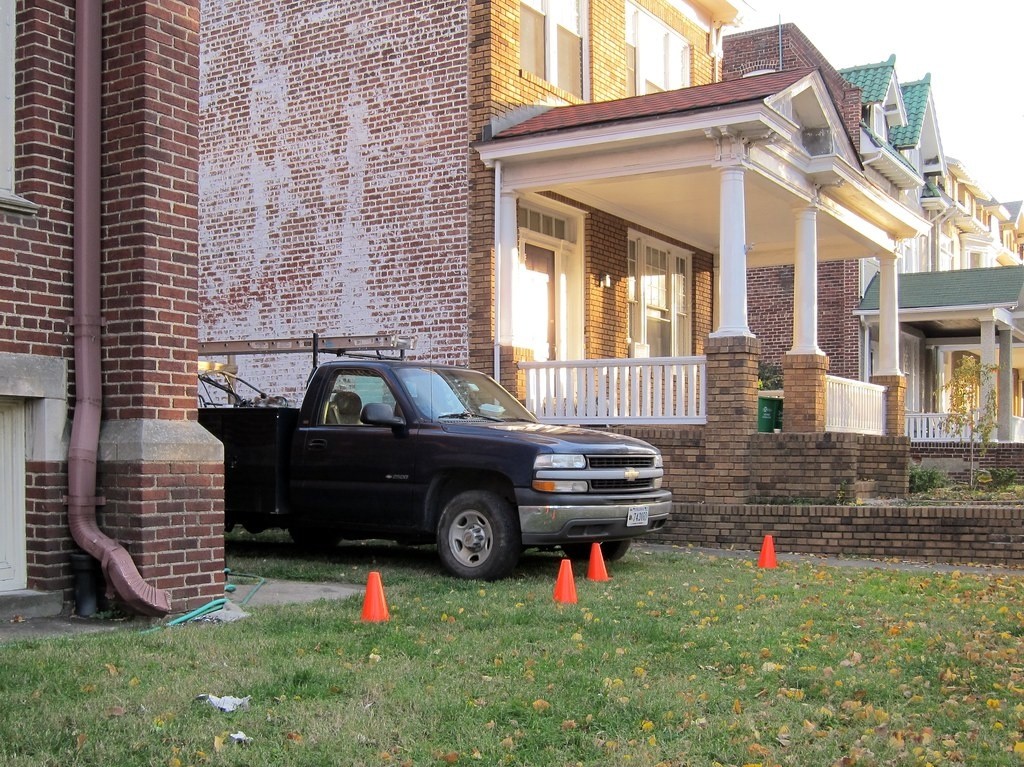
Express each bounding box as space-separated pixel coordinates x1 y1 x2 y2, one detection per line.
361 402 404 425
326 391 365 425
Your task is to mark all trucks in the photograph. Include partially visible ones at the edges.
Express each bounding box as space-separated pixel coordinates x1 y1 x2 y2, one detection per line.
198 354 665 578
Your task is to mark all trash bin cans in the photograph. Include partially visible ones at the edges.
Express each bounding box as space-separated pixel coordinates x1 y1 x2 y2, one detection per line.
758 396 782 433
